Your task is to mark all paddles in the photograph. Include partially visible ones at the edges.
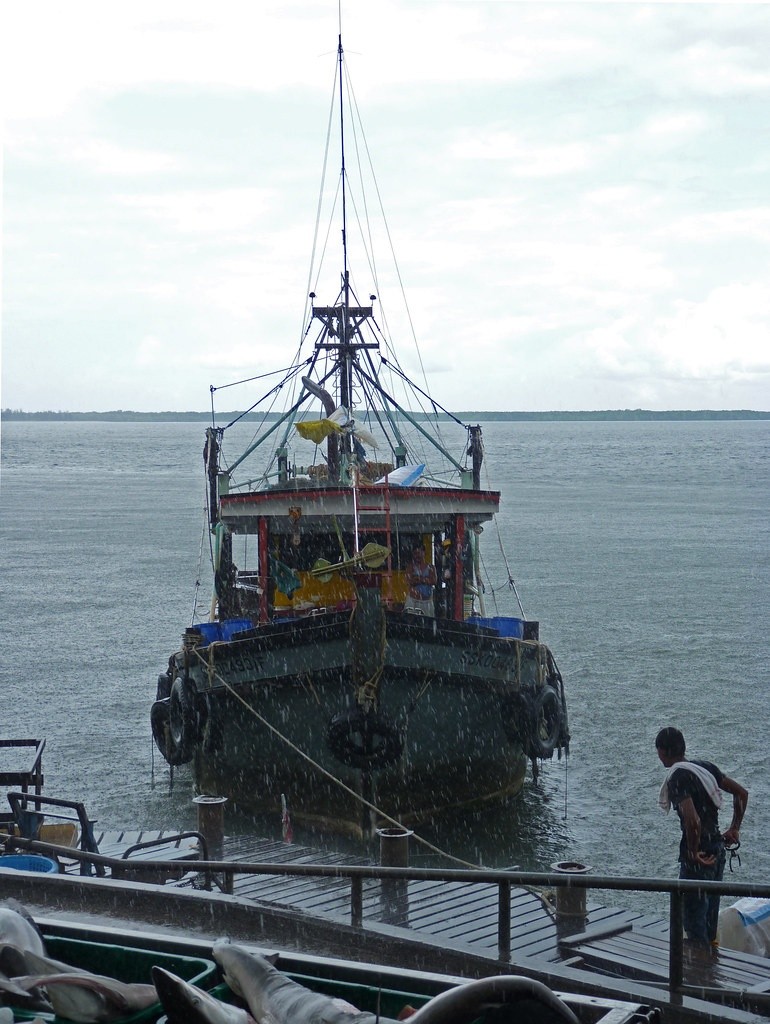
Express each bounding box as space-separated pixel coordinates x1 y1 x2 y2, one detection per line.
311 540 392 585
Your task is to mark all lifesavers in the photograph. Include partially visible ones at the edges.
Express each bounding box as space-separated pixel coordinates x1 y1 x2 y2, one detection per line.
503 673 569 759
325 709 406 768
150 671 197 763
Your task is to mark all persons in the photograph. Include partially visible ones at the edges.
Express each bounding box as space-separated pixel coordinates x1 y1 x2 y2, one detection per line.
655 727 748 940
405 544 437 616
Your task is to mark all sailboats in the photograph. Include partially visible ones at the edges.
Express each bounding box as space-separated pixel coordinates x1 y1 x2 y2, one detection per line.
148 0 571 844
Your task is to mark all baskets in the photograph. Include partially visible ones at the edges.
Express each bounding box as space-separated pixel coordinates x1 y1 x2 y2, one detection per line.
0 855 59 873
